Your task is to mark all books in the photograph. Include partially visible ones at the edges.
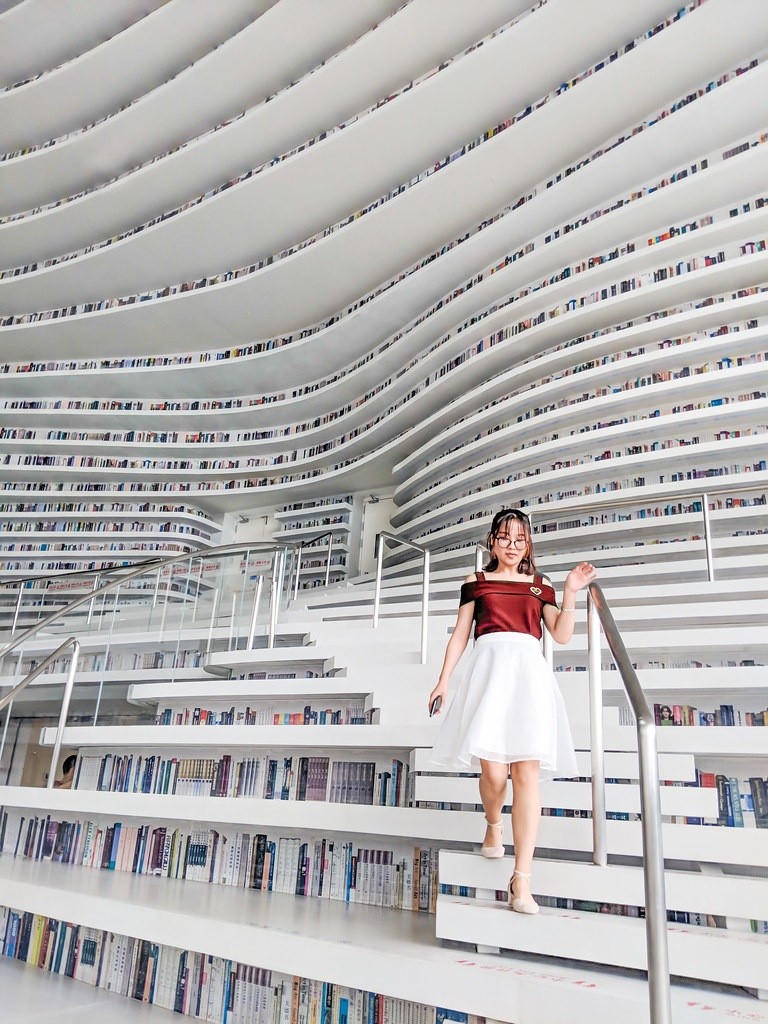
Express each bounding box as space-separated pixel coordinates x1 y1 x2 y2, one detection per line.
0 0 767 1024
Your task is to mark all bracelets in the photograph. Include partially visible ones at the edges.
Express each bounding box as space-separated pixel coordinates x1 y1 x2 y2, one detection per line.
560 606 574 612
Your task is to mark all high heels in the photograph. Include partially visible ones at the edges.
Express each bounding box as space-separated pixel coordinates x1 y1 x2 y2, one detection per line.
508 870 539 915
480 815 505 857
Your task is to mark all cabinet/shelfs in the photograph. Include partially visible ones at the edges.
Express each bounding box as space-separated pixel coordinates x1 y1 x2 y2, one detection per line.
0 1 767 1024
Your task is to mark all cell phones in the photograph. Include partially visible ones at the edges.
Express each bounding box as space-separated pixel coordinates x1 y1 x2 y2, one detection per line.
431 695 442 717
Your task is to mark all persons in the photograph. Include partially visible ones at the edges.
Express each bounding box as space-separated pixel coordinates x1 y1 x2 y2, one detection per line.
427 510 595 916
660 706 673 726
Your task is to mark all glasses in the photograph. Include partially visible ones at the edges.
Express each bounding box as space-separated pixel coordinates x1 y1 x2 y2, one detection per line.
493 535 530 550
